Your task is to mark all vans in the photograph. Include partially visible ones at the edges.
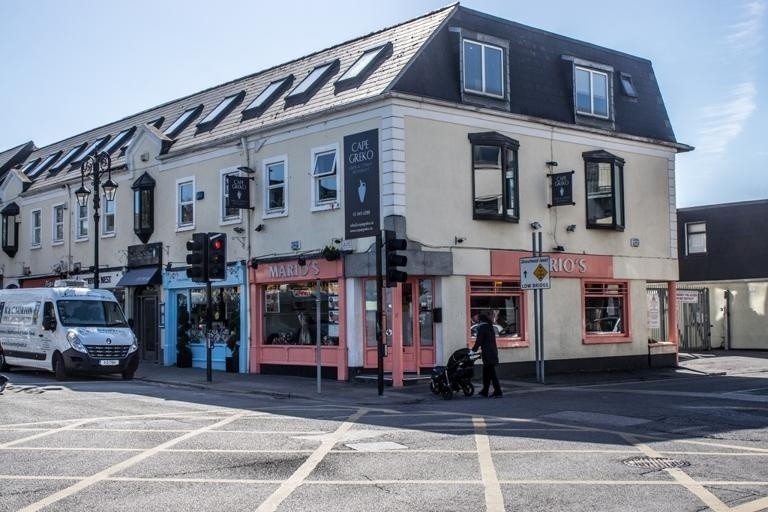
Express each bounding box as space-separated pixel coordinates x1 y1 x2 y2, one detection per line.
0 287 141 383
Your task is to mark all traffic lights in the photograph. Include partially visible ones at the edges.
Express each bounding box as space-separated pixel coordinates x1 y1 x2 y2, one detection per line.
185 231 207 283
383 228 408 289
205 230 228 282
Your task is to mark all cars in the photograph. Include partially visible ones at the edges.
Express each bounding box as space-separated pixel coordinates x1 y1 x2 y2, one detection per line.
585 317 622 335
469 321 518 338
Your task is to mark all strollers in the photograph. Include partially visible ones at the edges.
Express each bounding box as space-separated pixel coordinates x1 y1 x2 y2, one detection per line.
426 345 479 400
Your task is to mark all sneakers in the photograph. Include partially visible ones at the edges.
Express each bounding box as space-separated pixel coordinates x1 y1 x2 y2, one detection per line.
478 391 489 397
489 390 503 398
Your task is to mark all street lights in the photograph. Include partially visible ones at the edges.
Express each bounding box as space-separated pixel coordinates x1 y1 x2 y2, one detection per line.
72 145 119 290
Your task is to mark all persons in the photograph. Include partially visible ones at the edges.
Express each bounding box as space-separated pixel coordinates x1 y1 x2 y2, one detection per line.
58 306 66 318
297 311 313 344
472 312 502 397
